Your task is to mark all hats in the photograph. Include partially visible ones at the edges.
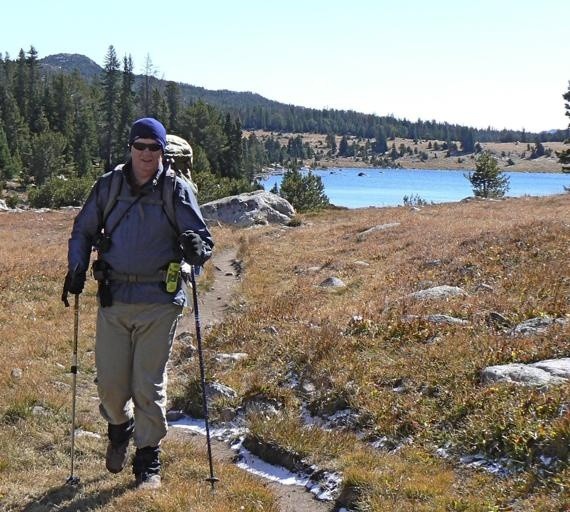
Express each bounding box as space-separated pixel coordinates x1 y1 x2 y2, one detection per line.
128 117 168 148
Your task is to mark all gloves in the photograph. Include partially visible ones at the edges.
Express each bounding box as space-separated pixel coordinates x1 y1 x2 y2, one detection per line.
65 272 89 295
176 228 206 266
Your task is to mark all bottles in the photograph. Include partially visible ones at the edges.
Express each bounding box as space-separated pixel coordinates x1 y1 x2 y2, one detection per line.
194 265 201 277
100 280 113 307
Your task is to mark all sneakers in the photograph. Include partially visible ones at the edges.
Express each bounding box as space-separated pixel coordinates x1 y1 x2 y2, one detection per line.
102 437 129 473
132 445 172 498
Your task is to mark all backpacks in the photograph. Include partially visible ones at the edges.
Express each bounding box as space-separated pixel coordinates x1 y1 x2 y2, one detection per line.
91 136 196 284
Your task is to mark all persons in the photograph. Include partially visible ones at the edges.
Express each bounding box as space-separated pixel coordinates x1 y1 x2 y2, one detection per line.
64 117 215 487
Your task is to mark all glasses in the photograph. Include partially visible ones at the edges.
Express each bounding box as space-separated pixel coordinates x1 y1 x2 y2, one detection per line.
129 140 162 152
